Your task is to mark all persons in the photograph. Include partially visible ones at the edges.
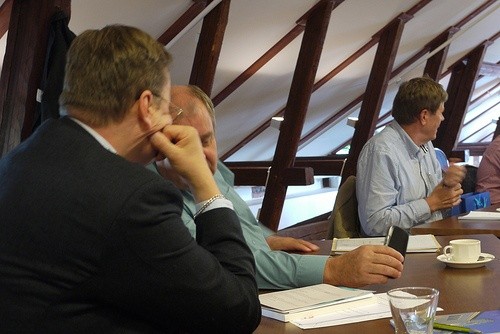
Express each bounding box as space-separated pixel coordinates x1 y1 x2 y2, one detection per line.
356 77 466 237
0 24 261 334
144 84 405 290
475 117 500 204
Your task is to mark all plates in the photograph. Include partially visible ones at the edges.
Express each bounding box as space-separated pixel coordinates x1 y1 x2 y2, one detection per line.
436 252 495 268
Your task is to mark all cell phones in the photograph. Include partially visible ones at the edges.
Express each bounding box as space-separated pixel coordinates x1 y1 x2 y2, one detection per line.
385 226 409 279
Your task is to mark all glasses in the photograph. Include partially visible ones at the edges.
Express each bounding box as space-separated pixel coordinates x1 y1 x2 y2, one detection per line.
136 87 183 121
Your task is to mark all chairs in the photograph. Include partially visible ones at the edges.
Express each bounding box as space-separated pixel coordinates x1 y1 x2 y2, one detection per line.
327 175 361 238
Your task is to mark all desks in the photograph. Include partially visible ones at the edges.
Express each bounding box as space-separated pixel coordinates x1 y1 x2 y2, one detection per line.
251 204 500 334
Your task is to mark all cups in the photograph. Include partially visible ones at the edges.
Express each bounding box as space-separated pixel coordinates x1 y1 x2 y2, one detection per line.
443 239 481 262
388 287 440 334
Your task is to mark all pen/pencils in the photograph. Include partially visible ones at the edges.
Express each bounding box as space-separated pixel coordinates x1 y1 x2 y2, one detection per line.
390 319 482 333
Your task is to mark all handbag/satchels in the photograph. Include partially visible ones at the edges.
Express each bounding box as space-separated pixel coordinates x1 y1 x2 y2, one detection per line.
450 191 490 215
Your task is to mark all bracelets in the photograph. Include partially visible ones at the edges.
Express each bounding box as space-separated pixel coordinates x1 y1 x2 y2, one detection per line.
193 192 225 221
